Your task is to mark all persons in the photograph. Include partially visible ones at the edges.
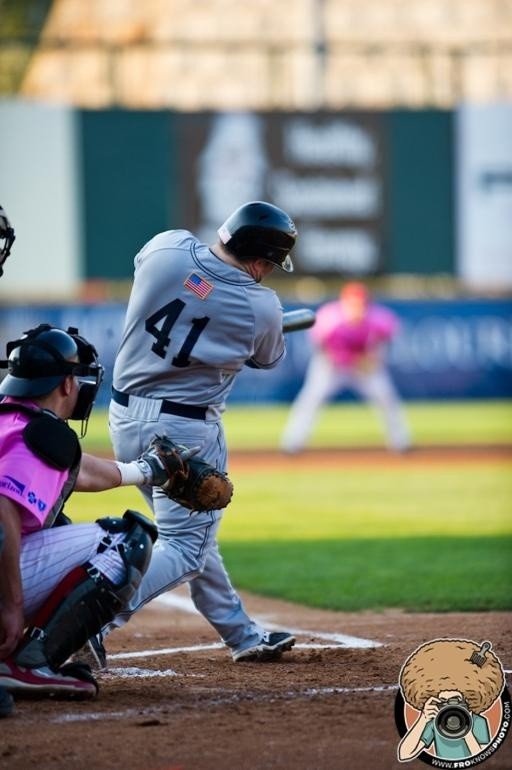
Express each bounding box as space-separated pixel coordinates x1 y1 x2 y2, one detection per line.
76 202 297 668
1 327 234 701
280 282 416 456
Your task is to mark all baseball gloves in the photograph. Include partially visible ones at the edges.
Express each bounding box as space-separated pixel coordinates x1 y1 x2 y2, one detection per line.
139 438 234 513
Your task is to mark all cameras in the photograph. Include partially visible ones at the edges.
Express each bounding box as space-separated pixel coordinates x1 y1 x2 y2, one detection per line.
434 698 475 740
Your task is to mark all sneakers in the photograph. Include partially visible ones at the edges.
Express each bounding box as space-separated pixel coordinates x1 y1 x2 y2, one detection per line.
0 643 100 705
229 628 300 665
70 629 112 675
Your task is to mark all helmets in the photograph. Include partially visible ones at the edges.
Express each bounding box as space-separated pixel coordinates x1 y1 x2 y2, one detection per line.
214 199 299 273
0 326 88 400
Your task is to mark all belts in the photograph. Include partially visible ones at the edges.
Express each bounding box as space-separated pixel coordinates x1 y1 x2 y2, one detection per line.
108 384 208 422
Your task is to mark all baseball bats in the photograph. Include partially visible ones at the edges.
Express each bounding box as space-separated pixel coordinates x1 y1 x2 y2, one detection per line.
279 309 317 333
